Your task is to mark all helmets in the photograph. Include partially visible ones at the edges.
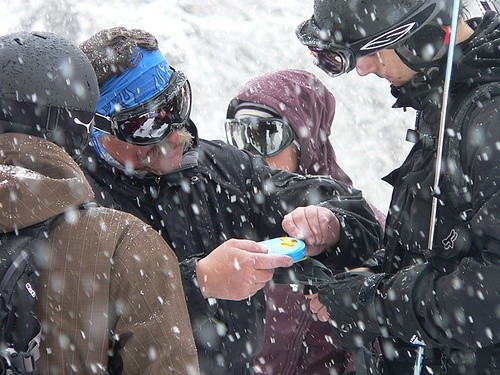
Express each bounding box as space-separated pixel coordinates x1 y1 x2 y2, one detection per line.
314 0 460 72
0 31 99 159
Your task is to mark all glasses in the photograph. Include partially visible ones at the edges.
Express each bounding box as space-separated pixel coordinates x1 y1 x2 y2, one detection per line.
296 0 446 77
225 117 300 158
93 66 192 146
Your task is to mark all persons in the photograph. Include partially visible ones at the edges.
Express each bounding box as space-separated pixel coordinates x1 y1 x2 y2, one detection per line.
75 27 385 375
225 69 386 375
0 31 201 375
295 0 500 375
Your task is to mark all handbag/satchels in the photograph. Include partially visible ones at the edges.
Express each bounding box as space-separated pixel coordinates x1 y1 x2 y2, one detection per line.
253 281 384 375
